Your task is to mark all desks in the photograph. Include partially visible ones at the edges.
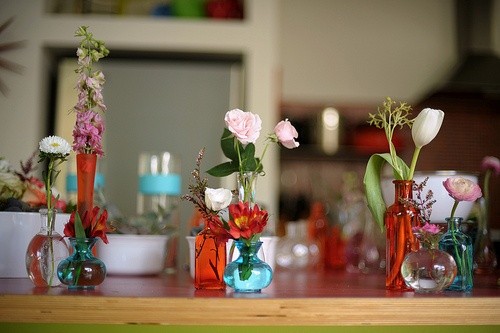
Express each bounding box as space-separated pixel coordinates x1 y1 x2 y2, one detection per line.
0 271 500 333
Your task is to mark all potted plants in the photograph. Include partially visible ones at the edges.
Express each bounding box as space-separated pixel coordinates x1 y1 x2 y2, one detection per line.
185 226 280 278
94 202 178 275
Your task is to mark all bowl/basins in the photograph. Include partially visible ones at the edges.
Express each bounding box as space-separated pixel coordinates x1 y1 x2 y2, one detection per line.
382 170 481 225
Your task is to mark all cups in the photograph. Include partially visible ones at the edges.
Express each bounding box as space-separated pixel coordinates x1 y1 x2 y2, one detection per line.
138 151 182 275
65 154 106 218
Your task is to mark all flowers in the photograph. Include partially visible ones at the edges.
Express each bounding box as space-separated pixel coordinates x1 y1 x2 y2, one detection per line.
181 107 300 281
68 23 110 170
59 206 118 284
38 134 73 287
361 96 445 233
481 155 500 200
398 176 444 275
442 177 483 272
0 149 77 212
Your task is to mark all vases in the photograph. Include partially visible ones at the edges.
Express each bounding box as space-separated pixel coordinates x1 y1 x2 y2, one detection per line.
70 154 97 257
385 179 497 294
0 212 108 289
222 241 273 293
194 214 226 291
